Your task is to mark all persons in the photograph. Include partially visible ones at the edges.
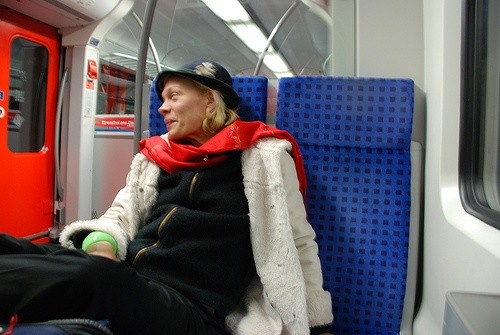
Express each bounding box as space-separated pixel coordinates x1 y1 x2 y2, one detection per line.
1 61 335 335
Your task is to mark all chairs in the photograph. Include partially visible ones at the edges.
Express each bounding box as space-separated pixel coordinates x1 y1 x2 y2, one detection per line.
147 76 427 335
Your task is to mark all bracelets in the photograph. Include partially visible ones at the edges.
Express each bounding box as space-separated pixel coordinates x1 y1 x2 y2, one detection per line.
79 231 119 257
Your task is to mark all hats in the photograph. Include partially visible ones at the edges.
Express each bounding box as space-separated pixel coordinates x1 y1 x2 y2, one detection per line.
157 60 240 109
